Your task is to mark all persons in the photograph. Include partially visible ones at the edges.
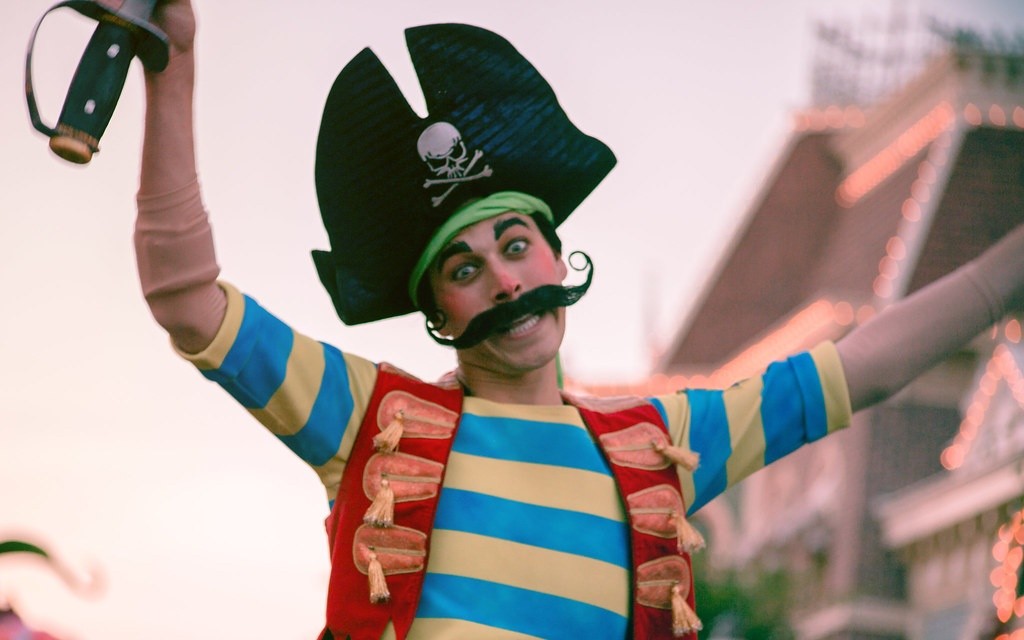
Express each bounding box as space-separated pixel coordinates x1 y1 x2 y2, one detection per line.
71 1 1024 639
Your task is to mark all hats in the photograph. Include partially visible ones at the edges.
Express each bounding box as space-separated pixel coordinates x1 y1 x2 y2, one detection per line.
311 22 618 325
